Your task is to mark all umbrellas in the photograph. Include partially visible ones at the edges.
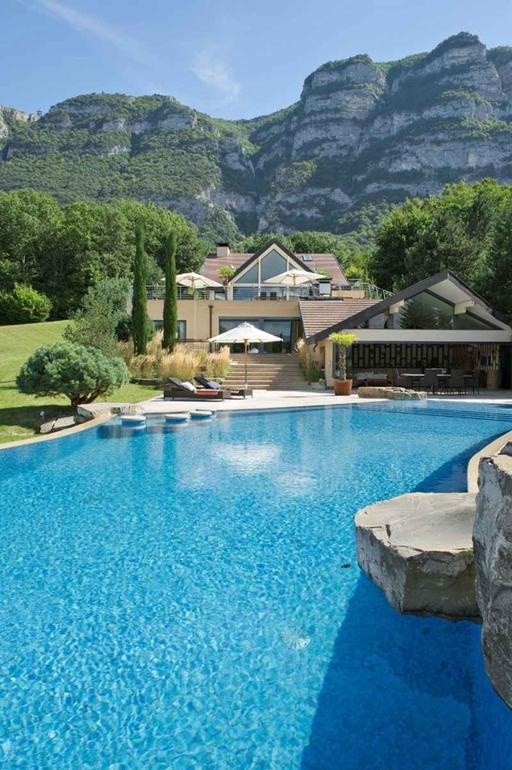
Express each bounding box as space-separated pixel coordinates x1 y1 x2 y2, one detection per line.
263 268 327 303
207 321 284 394
160 272 223 299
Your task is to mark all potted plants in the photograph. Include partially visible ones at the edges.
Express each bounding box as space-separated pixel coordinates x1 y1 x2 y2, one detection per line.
327 332 357 395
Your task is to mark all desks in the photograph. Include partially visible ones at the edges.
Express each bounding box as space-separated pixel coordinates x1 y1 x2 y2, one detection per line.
400 373 472 390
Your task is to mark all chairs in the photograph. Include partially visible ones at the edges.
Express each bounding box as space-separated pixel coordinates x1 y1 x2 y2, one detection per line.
164 376 230 402
443 368 465 396
418 372 437 394
195 374 253 398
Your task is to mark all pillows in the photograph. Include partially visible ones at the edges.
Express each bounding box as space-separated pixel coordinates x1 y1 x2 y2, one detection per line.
181 382 195 392
207 381 220 389
